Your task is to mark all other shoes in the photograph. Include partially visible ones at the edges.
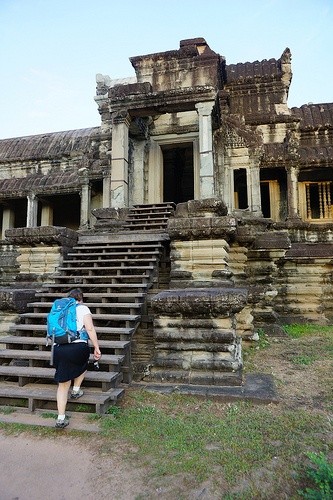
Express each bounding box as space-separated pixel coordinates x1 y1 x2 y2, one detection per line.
70 390 84 398
55 419 69 429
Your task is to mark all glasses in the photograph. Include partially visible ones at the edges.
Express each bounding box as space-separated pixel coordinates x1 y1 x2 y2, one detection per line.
94 360 100 370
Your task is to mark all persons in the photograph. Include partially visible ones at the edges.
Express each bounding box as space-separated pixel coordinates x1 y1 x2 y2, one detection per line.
52 288 101 428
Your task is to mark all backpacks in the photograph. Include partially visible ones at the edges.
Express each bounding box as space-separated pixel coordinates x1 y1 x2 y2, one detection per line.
47 298 89 344
